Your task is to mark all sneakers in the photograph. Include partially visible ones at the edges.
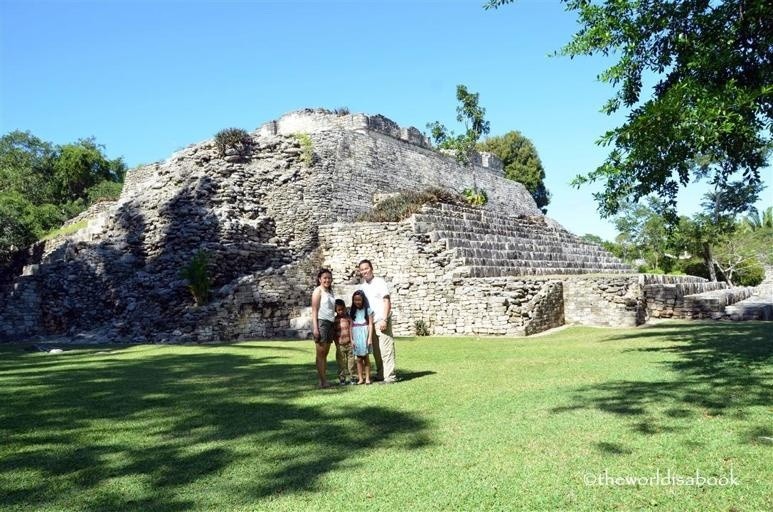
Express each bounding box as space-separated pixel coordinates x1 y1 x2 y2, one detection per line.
357 379 364 384
339 381 345 386
374 376 397 384
350 379 356 385
319 382 337 387
365 379 371 385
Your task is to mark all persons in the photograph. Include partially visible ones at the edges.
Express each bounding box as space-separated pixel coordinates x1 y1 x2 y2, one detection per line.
347 290 374 384
311 269 336 388
330 298 357 387
353 258 398 384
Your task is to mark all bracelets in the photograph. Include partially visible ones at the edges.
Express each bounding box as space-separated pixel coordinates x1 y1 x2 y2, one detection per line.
383 319 388 323
